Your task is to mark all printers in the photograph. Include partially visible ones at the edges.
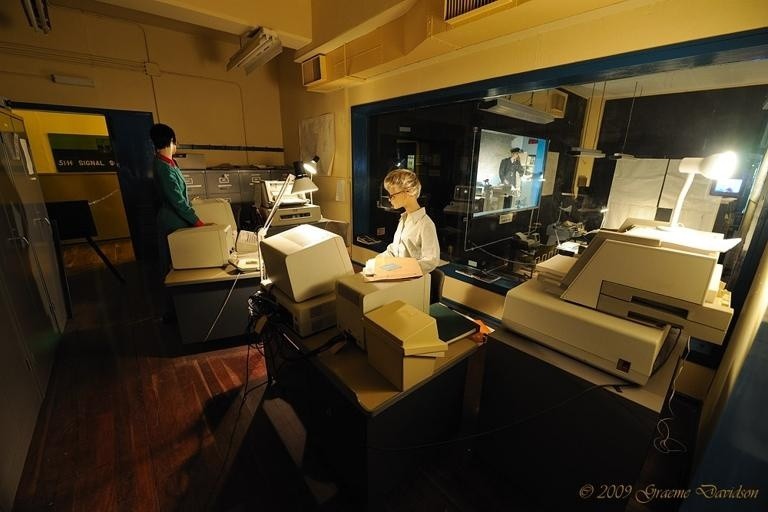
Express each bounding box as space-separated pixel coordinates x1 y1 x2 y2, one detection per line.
254 179 321 226
501 217 734 386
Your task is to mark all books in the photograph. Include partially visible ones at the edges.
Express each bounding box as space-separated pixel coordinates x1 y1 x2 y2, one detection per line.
429 301 481 345
534 253 579 296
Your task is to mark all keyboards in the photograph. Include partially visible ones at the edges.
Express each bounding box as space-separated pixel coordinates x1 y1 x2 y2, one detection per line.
237 229 258 251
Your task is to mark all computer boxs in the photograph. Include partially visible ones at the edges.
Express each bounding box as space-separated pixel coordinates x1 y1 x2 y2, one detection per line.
260 279 337 337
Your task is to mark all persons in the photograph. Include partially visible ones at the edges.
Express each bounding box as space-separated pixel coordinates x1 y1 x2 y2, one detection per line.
374 166 441 275
499 147 528 195
149 123 219 325
568 186 605 243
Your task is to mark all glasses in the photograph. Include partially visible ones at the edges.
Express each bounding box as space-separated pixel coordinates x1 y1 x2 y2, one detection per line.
389 190 405 200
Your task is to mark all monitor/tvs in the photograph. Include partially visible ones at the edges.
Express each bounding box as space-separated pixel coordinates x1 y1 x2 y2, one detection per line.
190 197 237 231
260 223 355 303
